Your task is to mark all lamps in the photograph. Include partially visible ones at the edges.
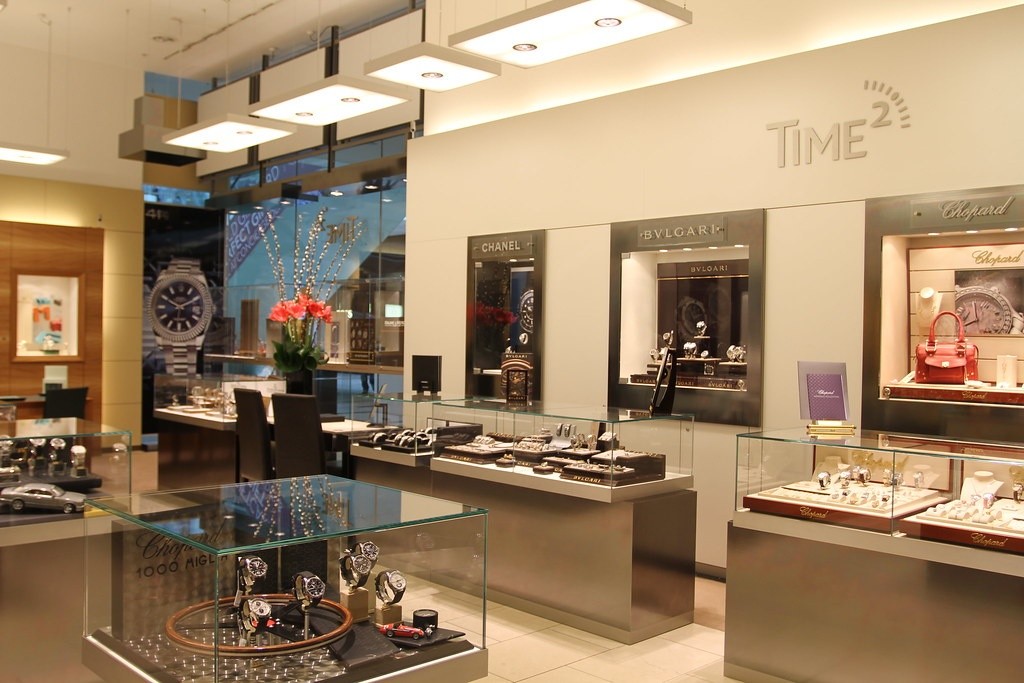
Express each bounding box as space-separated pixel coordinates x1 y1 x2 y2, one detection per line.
162 0 694 154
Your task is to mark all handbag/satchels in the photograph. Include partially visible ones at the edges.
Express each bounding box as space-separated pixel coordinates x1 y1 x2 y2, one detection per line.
914 312 978 385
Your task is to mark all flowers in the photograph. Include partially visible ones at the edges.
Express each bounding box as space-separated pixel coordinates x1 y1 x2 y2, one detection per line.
467 302 516 351
266 293 334 374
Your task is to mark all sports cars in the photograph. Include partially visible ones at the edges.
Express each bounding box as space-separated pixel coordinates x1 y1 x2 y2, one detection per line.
379 620 425 640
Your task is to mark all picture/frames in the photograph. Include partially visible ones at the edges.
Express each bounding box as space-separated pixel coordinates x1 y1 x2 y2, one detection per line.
10 266 85 362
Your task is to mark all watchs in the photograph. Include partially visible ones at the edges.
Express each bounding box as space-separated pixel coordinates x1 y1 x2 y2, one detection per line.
243 597 272 636
697 321 707 335
351 541 380 569
374 568 407 606
649 348 661 360
663 329 673 347
956 282 1023 335
983 493 995 509
913 471 924 489
841 471 851 488
726 345 746 361
147 255 216 374
0 434 86 467
373 428 436 446
1012 483 1023 502
893 471 904 489
291 571 326 609
852 465 872 483
473 423 627 471
684 341 698 358
818 471 830 488
238 554 268 594
341 553 372 589
517 288 533 332
883 468 893 486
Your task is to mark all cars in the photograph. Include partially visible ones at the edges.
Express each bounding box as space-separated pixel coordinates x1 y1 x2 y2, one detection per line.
0 482 88 515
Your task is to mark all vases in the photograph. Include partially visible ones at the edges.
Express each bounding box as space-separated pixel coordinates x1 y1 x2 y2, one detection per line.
285 369 314 396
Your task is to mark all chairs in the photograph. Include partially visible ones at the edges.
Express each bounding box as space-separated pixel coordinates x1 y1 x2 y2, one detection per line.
233 387 276 483
271 392 325 480
